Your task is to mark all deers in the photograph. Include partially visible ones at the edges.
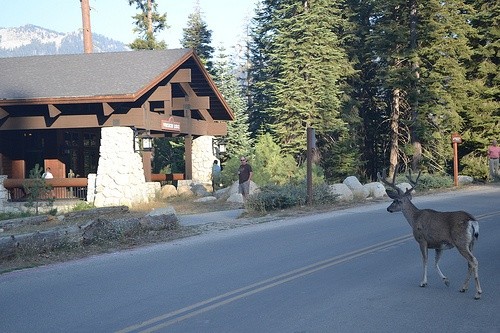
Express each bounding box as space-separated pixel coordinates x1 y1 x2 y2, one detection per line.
376 164 483 301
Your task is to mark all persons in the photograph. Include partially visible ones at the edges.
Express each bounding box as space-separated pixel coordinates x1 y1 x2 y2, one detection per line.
487 139 500 182
42 167 54 178
238 156 252 200
67 169 95 201
212 159 220 173
